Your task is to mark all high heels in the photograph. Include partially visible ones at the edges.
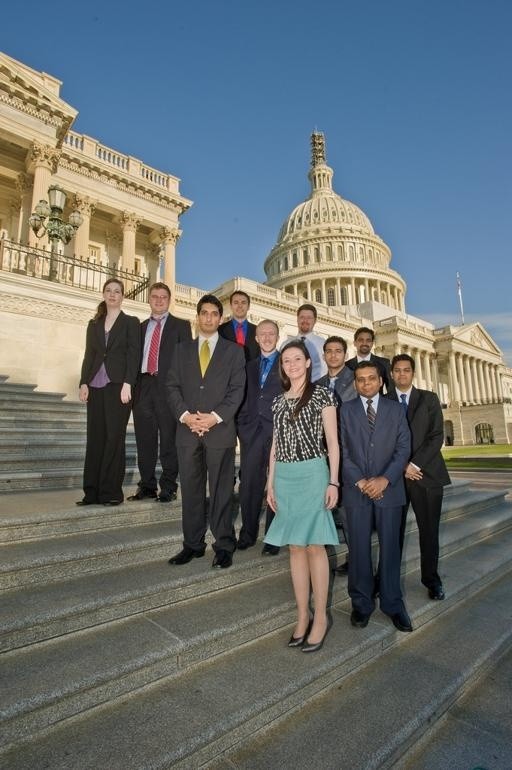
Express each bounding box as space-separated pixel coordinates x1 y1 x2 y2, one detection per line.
288 614 330 652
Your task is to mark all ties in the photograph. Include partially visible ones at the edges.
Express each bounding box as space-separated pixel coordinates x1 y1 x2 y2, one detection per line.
301 336 305 341
236 323 245 347
329 377 338 391
147 319 160 372
259 359 267 388
366 399 375 432
400 394 407 414
200 340 210 378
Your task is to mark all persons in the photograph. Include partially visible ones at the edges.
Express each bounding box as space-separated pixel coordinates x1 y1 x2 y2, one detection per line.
312 327 395 578
165 293 247 569
75 278 142 506
372 354 450 600
281 304 328 385
263 342 340 653
219 291 258 347
232 319 288 555
127 282 193 503
339 362 413 632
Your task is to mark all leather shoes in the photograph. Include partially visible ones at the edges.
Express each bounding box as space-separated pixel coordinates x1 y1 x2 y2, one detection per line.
392 610 412 632
169 548 204 564
429 585 444 600
333 563 350 576
351 609 368 627
77 487 176 506
262 544 280 556
212 540 254 568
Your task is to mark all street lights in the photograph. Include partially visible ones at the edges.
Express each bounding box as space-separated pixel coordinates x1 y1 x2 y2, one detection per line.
28 183 84 284
